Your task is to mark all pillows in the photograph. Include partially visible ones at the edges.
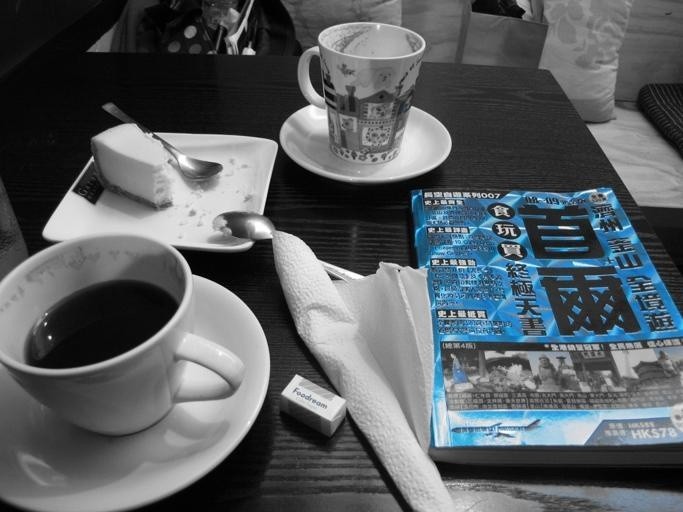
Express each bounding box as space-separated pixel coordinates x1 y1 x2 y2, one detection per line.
283 0 635 124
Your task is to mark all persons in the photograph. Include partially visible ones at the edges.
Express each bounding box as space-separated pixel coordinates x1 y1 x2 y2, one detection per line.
445 353 479 392
535 353 618 393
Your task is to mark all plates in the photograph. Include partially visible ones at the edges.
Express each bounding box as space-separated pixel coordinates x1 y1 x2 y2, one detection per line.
279 96 452 187
0 274 271 508
41 133 279 252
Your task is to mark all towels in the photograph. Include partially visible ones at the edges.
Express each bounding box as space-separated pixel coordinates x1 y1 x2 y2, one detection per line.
272 229 458 512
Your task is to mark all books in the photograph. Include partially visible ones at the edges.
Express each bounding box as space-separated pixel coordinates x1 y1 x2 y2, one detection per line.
411 185 683 466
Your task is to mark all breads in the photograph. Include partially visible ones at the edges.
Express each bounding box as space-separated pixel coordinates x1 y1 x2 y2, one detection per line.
90 121 173 210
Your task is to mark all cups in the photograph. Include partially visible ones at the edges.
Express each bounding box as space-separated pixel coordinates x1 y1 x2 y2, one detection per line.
0 232 245 438
297 23 426 166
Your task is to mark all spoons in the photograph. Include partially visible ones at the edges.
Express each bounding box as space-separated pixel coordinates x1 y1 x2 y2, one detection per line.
214 209 368 282
99 98 223 185
